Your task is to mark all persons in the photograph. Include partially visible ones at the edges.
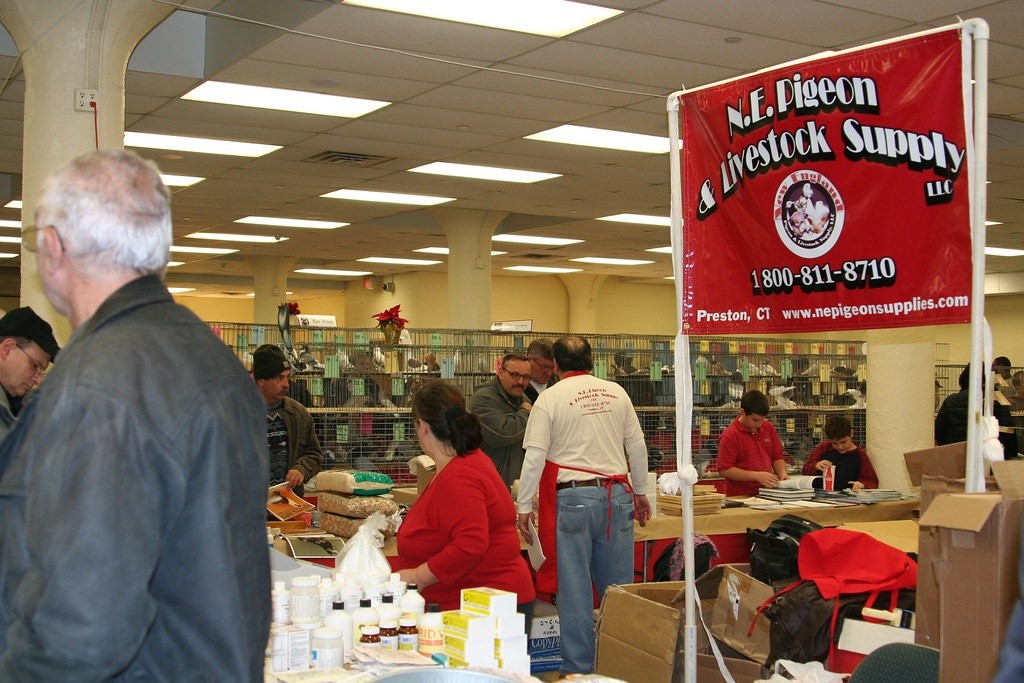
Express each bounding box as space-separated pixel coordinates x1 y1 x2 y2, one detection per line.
522 338 559 406
0 306 63 442
424 353 442 379
935 356 1024 458
391 378 535 613
469 353 533 492
716 391 786 495
803 417 878 490
514 336 652 676
247 344 323 521
0 150 274 683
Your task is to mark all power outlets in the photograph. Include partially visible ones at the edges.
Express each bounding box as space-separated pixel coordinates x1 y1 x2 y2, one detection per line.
75 87 99 112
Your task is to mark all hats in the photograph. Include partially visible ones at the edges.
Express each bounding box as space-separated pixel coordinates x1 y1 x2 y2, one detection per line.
0 306 61 364
253 344 291 381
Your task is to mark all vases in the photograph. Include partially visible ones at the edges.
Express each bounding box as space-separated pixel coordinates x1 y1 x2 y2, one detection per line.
383 325 403 345
289 314 300 326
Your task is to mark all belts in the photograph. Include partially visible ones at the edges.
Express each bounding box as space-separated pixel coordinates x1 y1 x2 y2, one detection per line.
556 478 618 490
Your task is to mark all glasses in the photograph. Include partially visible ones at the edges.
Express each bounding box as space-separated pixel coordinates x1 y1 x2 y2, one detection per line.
502 367 533 382
16 343 46 378
531 356 554 372
21 225 65 253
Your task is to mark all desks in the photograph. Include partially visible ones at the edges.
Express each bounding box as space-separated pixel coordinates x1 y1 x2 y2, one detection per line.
274 370 916 613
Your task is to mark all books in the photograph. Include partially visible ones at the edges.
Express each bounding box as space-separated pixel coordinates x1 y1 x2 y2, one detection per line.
758 475 821 502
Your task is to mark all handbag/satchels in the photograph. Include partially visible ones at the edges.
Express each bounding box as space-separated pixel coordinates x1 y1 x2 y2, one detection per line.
827 604 915 674
747 513 826 580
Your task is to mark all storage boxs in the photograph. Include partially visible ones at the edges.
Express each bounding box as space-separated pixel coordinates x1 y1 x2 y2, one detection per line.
388 437 1024 683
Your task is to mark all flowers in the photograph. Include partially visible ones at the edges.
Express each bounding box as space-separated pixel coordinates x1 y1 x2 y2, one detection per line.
278 302 301 315
370 304 408 330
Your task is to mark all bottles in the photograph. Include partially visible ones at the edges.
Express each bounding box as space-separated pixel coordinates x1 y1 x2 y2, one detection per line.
268 572 444 674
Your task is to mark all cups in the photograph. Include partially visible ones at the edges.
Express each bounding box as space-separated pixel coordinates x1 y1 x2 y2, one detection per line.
823 465 836 490
